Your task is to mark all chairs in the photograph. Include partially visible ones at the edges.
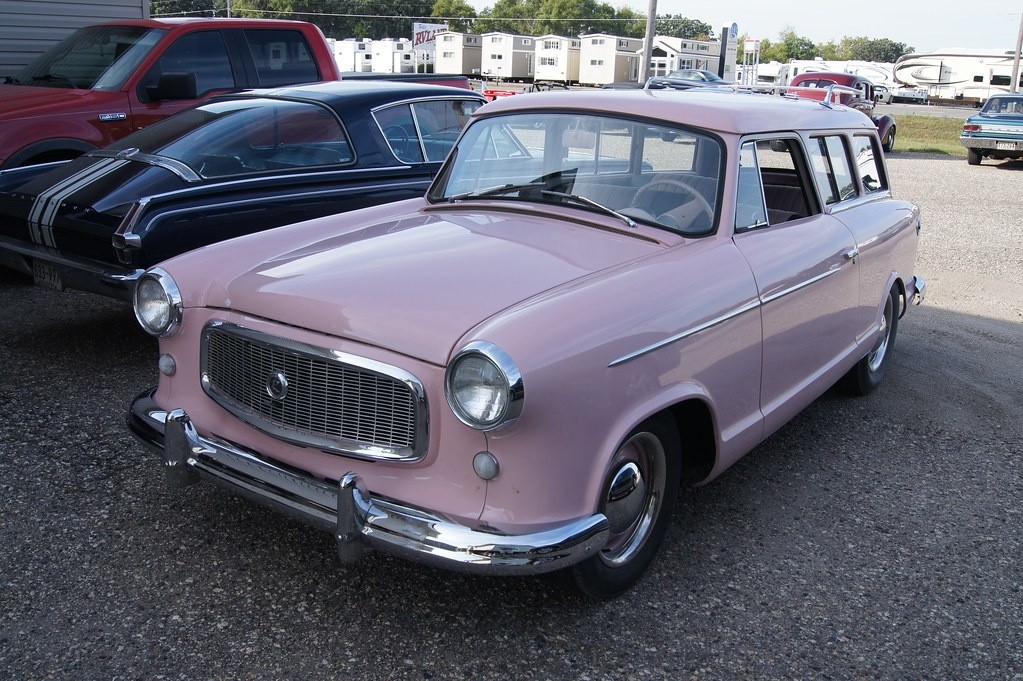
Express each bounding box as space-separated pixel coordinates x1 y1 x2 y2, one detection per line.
282 60 314 70
986 104 1001 113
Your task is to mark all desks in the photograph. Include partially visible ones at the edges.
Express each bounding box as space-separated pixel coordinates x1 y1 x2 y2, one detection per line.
485 89 523 93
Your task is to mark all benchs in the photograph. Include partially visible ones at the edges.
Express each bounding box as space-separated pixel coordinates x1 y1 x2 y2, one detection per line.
630 173 807 215
561 182 802 225
267 147 343 165
387 138 455 162
484 94 515 101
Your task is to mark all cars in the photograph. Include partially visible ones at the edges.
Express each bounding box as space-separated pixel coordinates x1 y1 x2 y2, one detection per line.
663 69 734 86
124 90 925 594
0 79 653 304
769 73 897 153
958 94 1023 166
476 78 751 144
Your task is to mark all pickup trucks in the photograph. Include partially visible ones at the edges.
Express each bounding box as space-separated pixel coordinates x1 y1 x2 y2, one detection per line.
872 85 894 105
0 18 468 279
